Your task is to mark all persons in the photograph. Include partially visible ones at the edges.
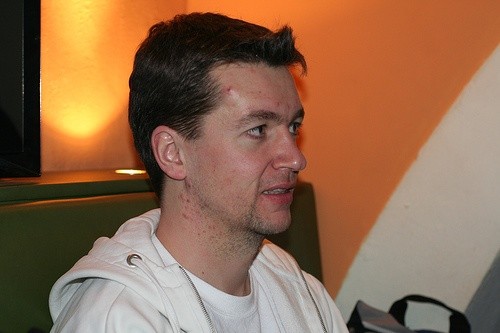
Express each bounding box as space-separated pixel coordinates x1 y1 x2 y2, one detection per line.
48 11 354 333
